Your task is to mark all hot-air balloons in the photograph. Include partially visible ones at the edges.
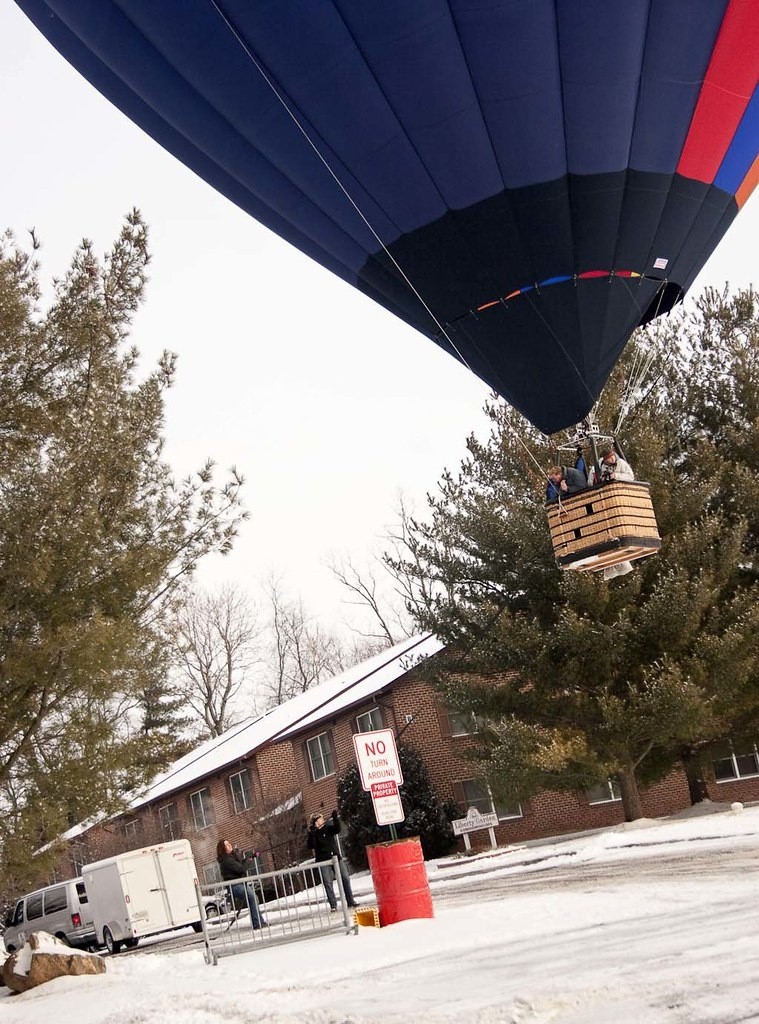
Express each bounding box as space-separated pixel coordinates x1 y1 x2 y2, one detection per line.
14 0 759 584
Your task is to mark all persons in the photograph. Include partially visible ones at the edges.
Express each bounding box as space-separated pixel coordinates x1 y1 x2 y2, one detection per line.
587 449 635 486
306 810 360 912
548 465 587 499
216 840 267 930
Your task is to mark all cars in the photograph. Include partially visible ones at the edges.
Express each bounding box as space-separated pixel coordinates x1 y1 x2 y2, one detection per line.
213 878 279 912
203 895 232 919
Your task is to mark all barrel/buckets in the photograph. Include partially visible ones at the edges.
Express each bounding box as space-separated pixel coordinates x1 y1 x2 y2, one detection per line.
365 836 433 926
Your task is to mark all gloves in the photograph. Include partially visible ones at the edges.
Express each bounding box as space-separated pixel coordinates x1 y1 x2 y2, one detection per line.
310 826 316 832
332 809 338 819
253 851 260 858
246 858 252 864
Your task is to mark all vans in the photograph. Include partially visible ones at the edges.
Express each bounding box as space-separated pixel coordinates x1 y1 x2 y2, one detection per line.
3 877 102 954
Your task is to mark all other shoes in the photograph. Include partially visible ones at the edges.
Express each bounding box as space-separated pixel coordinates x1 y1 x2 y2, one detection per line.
348 902 360 907
331 907 337 913
254 922 271 929
